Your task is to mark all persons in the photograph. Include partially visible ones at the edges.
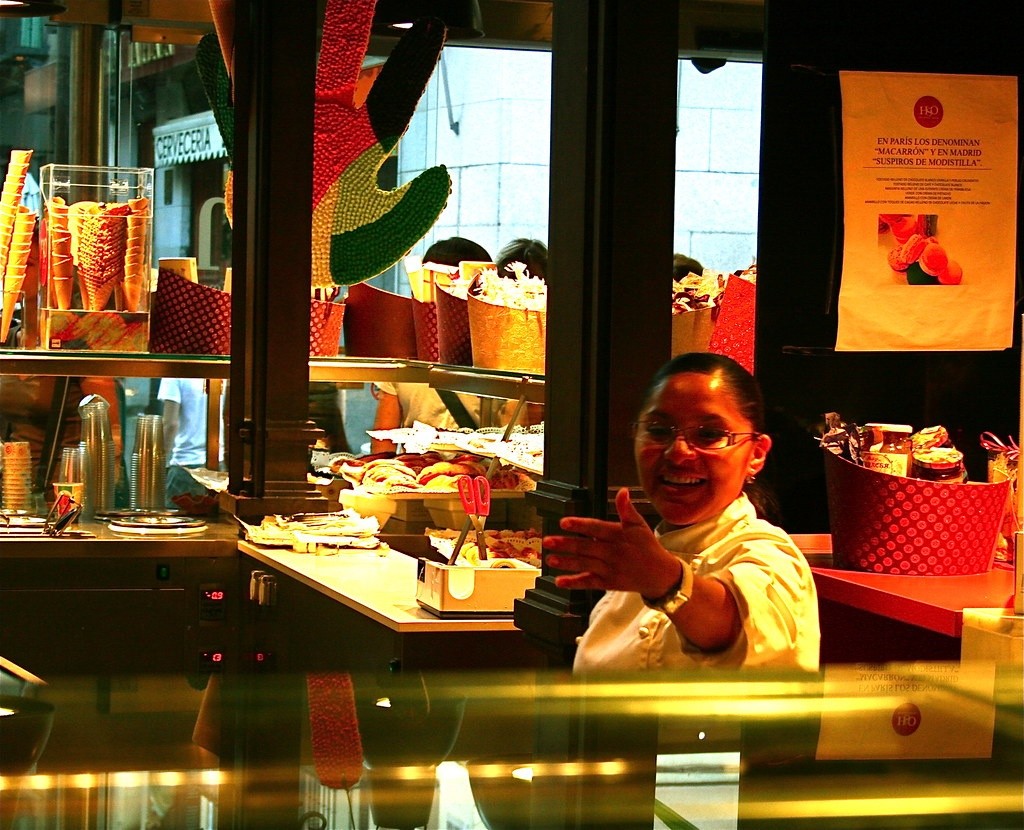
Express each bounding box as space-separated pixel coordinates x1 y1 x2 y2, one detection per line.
542 352 821 680
371 237 493 454
157 378 227 508
492 239 548 431
673 251 704 281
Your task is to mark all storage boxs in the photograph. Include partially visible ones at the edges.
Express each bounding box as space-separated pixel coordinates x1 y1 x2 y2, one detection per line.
343 282 418 360
148 267 231 354
38 308 149 352
467 271 546 374
410 291 439 362
310 298 346 357
433 280 474 367
821 443 1012 577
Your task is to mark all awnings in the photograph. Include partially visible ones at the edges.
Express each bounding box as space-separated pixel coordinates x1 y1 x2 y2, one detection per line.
152 110 228 168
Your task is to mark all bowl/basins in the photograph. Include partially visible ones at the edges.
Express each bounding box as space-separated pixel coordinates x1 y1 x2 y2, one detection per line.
2 441 33 511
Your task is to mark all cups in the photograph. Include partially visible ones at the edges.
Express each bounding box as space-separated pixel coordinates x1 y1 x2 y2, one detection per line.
54 402 115 523
129 413 167 511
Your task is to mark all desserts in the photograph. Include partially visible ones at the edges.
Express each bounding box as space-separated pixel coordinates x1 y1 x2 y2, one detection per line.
450 527 541 568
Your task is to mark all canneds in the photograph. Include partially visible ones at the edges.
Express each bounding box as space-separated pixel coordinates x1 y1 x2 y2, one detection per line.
862 424 969 485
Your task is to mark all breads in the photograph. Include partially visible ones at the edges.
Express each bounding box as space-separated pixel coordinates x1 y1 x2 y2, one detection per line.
327 451 536 491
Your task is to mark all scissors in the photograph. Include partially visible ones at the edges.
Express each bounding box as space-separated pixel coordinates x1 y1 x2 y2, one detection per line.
457 475 491 560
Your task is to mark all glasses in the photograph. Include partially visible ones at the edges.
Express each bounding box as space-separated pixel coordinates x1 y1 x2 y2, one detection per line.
632 417 762 452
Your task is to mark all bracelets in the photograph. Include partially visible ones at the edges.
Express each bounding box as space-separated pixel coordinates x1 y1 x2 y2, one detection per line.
640 557 691 612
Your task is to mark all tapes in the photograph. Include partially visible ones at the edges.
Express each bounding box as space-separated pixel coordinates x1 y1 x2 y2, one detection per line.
490 560 517 568
